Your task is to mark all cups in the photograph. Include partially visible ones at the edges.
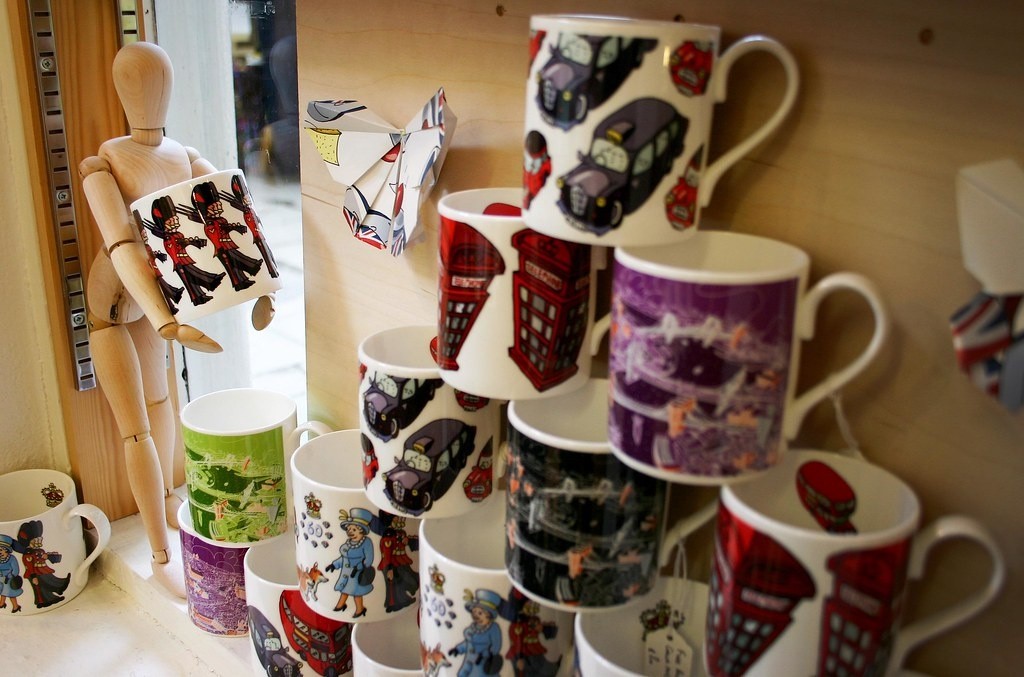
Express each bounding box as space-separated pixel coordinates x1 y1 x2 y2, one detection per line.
129 169 284 326
178 15 1004 677
0 469 111 615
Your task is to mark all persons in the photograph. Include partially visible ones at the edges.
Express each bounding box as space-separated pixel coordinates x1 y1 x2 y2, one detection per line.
76 40 275 599
